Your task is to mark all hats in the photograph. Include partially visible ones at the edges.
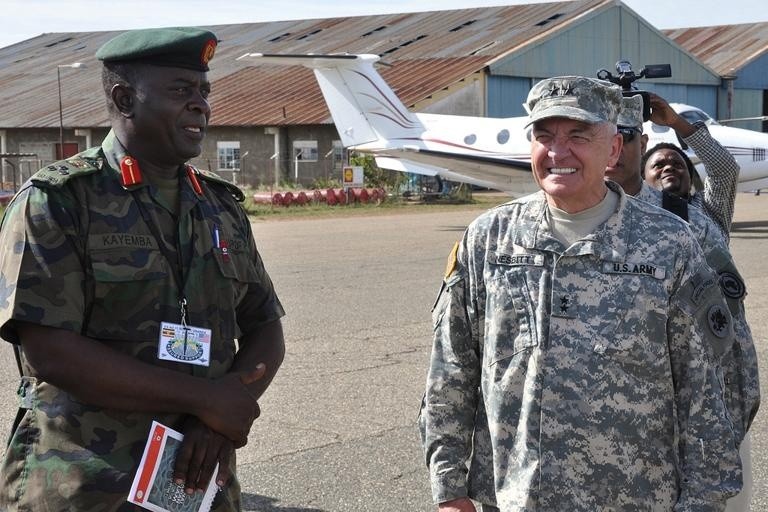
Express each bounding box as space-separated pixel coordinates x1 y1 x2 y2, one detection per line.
616 94 644 128
523 75 623 130
95 27 218 72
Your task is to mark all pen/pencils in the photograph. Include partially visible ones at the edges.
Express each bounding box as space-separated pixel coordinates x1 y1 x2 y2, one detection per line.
213 223 220 248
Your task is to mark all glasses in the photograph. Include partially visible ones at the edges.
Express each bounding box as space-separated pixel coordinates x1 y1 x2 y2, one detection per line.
617 127 643 144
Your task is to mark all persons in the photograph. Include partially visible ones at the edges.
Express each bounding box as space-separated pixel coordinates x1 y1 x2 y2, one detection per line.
419 74 746 510
605 93 762 447
0 28 287 511
639 90 742 250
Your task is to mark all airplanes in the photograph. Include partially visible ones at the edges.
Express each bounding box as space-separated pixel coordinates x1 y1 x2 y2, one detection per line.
235 51 768 204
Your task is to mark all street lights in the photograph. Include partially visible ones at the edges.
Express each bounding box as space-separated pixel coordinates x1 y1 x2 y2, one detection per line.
56 61 87 161
